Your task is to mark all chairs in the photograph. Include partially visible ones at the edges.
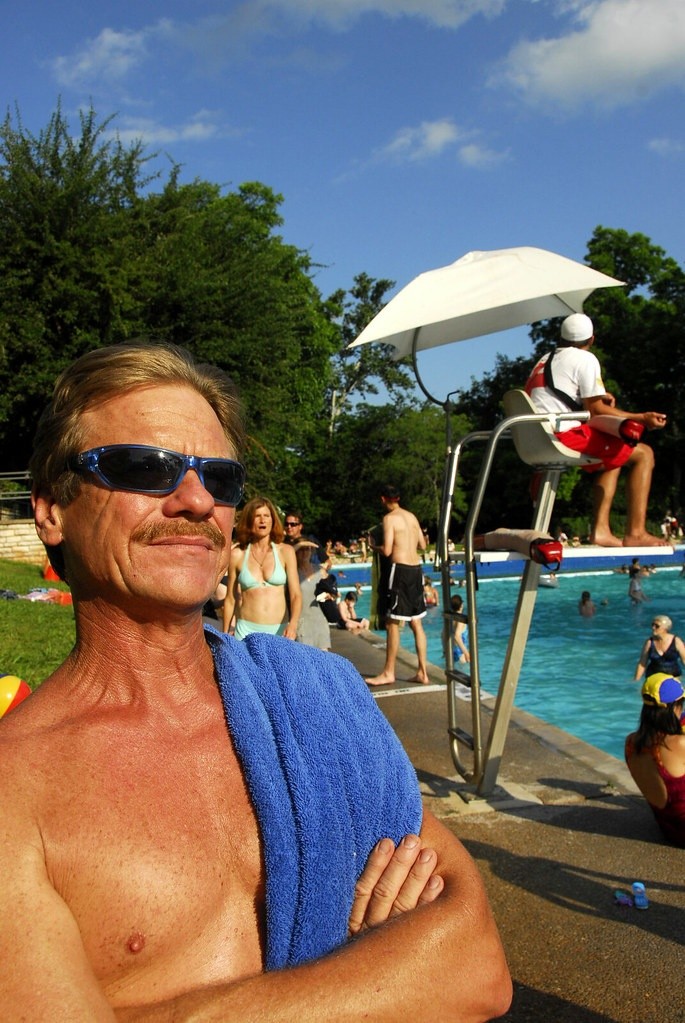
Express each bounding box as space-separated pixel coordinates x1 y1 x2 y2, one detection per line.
500 387 602 467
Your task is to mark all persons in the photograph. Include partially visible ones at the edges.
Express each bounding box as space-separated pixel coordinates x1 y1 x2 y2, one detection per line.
44 555 60 580
555 525 591 548
679 563 685 581
624 672 685 828
524 312 669 547
629 572 651 607
224 498 305 641
449 576 467 587
600 599 608 606
364 484 427 686
613 558 656 577
282 513 370 652
578 591 596 618
442 594 470 664
0 341 512 1023
423 576 440 609
660 515 683 544
634 615 685 681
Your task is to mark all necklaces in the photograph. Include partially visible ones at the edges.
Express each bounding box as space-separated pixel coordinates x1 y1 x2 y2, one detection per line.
252 548 270 568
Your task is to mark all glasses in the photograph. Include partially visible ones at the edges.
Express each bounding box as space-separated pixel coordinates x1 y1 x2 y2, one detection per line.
651 623 665 629
284 522 301 527
47 443 245 506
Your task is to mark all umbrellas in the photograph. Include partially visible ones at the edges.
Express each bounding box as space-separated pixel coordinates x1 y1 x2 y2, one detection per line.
345 245 629 410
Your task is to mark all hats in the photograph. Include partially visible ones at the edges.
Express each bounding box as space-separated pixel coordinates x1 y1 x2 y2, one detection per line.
642 673 685 707
561 313 594 341
